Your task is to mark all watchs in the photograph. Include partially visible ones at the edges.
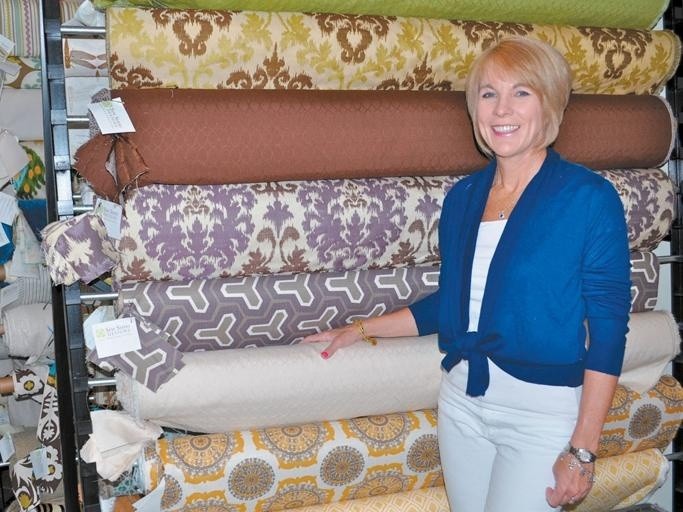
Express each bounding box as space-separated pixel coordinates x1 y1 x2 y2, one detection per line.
565 442 597 466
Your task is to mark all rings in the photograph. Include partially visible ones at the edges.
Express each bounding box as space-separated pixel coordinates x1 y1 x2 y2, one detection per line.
570 496 578 507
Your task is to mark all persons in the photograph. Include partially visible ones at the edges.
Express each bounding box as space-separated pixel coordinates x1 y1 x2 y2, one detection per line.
294 33 631 512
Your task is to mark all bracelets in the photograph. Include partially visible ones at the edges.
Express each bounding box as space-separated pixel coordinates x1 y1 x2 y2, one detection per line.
351 318 377 348
560 450 593 485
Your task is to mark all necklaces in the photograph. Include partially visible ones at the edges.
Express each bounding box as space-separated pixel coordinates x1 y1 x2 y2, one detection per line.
496 209 505 221
